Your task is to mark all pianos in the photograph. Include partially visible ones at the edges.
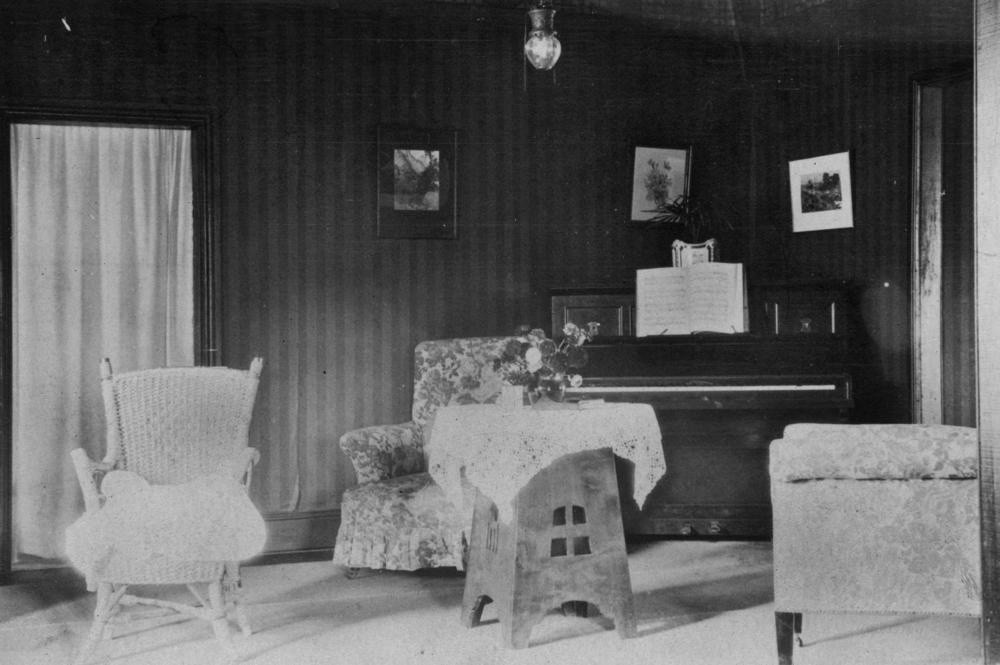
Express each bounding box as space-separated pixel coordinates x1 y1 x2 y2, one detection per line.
544 276 856 540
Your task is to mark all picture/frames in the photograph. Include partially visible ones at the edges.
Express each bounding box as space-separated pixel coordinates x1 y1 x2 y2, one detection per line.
789 150 855 232
628 142 693 231
376 122 456 241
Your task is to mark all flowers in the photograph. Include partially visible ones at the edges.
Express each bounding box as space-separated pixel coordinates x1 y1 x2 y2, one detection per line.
493 321 601 397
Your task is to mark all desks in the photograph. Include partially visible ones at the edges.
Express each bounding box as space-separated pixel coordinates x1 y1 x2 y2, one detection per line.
430 400 667 650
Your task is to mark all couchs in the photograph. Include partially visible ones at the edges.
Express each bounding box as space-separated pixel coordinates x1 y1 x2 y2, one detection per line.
332 336 529 579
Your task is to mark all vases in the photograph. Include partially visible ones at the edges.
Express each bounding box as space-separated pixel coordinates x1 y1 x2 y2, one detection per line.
532 391 560 410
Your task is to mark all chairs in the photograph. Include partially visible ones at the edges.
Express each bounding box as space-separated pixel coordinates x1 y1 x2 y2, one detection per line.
72 357 263 663
767 423 980 665
